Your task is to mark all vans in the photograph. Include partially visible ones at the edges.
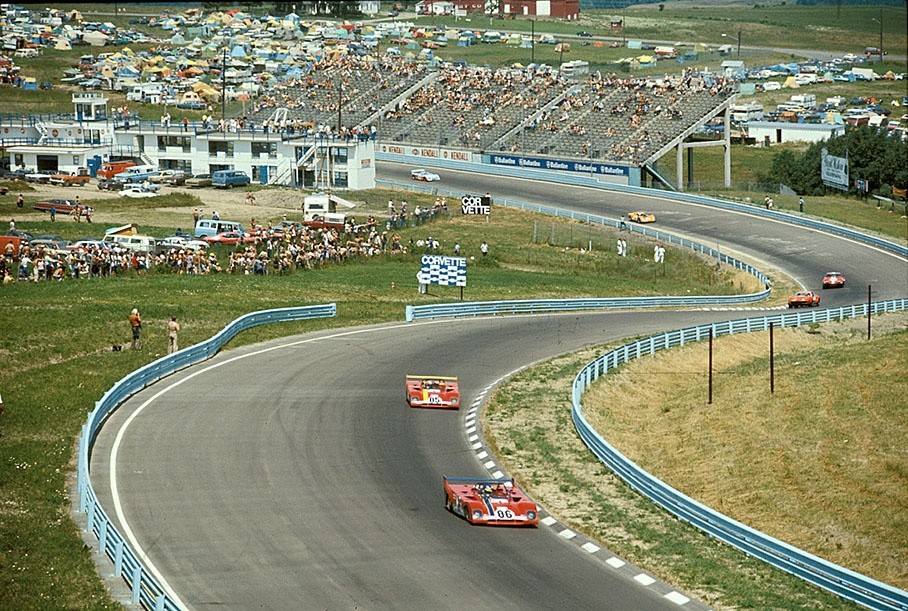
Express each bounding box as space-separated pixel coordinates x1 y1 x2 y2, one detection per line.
195 220 244 241
212 170 250 189
100 235 155 254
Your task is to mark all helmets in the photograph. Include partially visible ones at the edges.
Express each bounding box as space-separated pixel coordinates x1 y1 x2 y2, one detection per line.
425 380 432 389
476 484 491 495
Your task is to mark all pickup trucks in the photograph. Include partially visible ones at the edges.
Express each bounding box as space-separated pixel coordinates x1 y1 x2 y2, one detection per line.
300 194 356 230
49 171 90 187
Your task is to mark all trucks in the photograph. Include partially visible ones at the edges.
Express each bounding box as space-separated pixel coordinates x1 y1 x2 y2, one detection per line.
97 160 176 184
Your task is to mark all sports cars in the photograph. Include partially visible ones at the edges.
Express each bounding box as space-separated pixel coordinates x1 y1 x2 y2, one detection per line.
443 474 539 526
823 272 846 289
628 210 655 223
788 290 821 309
411 168 441 182
405 375 460 409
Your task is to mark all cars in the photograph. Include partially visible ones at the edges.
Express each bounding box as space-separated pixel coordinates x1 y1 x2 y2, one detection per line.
203 232 255 246
4 229 111 259
0 0 309 109
4 170 30 180
25 170 56 184
33 199 86 214
0 186 8 195
97 179 161 198
157 235 210 253
270 221 303 232
311 3 656 76
163 173 190 185
693 47 908 144
185 174 213 188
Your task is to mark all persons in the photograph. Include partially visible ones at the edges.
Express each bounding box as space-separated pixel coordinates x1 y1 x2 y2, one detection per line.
479 241 488 257
618 215 625 232
652 244 666 264
167 315 181 354
1 2 739 294
799 196 804 213
484 191 491 205
129 308 143 351
763 196 773 209
453 242 463 256
616 238 628 257
